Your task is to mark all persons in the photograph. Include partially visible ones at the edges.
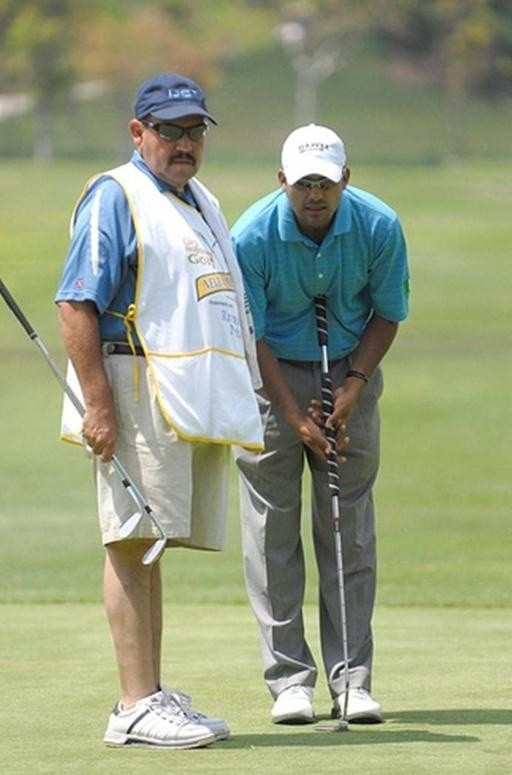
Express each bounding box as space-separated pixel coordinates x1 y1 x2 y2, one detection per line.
54 74 267 748
230 122 410 724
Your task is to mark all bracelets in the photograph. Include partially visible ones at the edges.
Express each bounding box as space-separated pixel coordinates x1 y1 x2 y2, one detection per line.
345 370 368 382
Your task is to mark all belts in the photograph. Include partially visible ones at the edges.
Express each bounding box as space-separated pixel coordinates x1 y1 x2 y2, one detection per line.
276 355 349 372
108 343 145 355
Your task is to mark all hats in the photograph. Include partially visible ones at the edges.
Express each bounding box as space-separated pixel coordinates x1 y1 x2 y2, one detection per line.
134 73 218 125
281 123 346 186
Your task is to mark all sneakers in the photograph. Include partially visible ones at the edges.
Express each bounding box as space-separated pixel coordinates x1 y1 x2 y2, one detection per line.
103 687 230 749
272 685 315 724
331 687 381 721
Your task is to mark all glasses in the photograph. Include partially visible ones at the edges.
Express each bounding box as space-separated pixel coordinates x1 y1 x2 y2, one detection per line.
294 177 335 192
141 119 208 142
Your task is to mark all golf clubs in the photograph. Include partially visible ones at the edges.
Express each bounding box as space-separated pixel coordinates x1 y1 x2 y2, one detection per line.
314 293 351 731
0 278 166 565
